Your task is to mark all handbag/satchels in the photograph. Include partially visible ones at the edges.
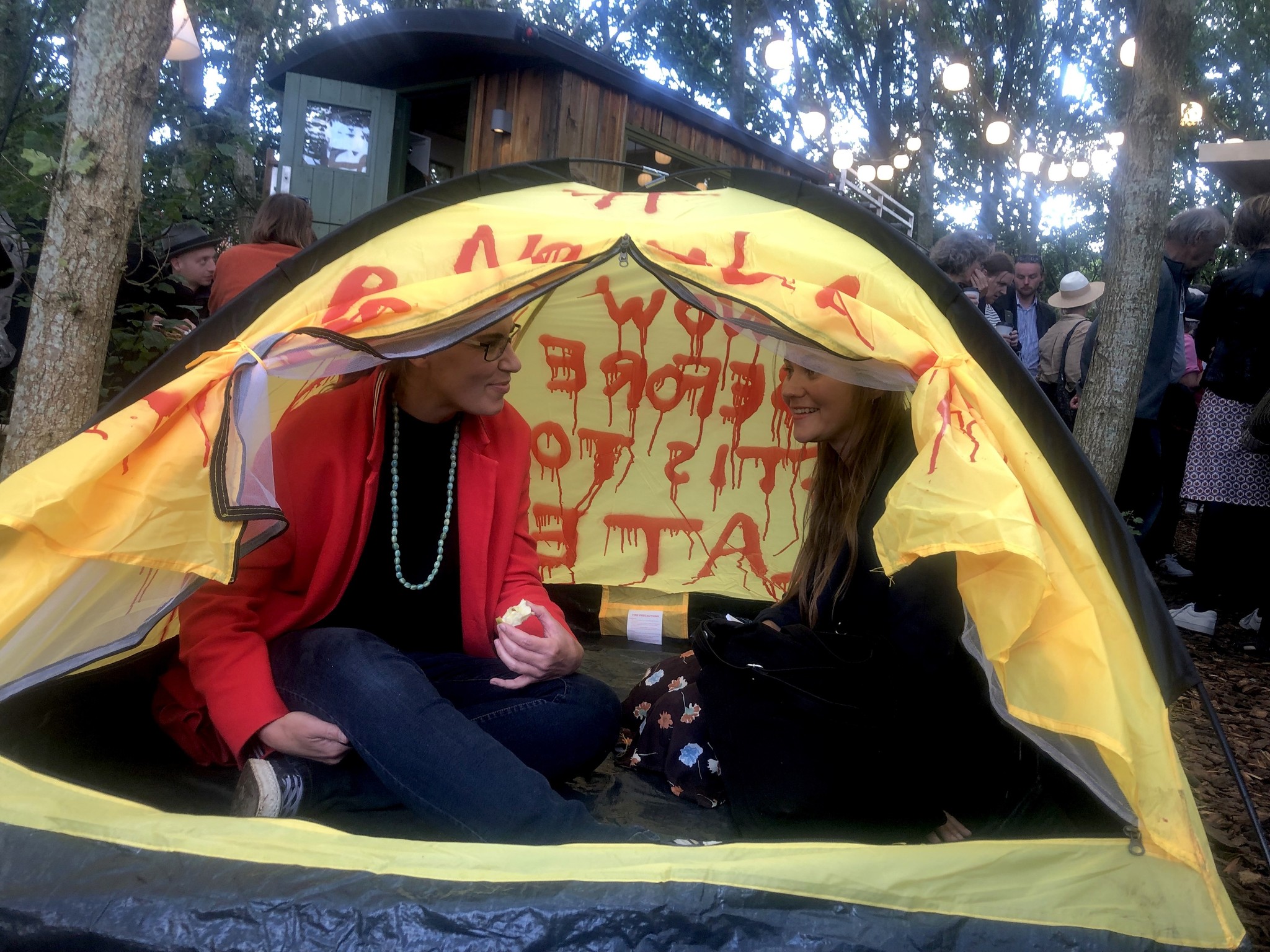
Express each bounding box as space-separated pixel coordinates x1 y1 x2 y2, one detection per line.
1050 378 1082 426
1241 388 1270 455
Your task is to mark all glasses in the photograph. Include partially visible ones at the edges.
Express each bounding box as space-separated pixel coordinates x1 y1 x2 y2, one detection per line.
296 195 310 207
1016 254 1044 273
462 322 521 362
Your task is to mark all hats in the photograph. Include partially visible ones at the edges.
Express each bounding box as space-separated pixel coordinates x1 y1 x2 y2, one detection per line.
158 219 224 262
1048 270 1106 308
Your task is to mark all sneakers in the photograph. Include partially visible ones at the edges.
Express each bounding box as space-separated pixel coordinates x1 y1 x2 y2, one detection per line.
1238 608 1262 633
1168 602 1217 636
670 838 723 846
1185 503 1204 515
1155 552 1193 577
230 759 304 818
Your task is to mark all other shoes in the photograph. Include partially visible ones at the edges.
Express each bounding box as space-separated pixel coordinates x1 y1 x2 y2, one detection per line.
1241 641 1270 661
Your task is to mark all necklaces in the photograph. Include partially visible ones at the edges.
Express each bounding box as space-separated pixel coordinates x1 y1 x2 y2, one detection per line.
389 385 462 591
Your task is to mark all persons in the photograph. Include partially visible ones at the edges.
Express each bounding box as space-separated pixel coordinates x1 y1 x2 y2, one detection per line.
924 195 1270 667
608 329 1082 846
151 301 722 846
143 219 223 338
207 192 315 318
